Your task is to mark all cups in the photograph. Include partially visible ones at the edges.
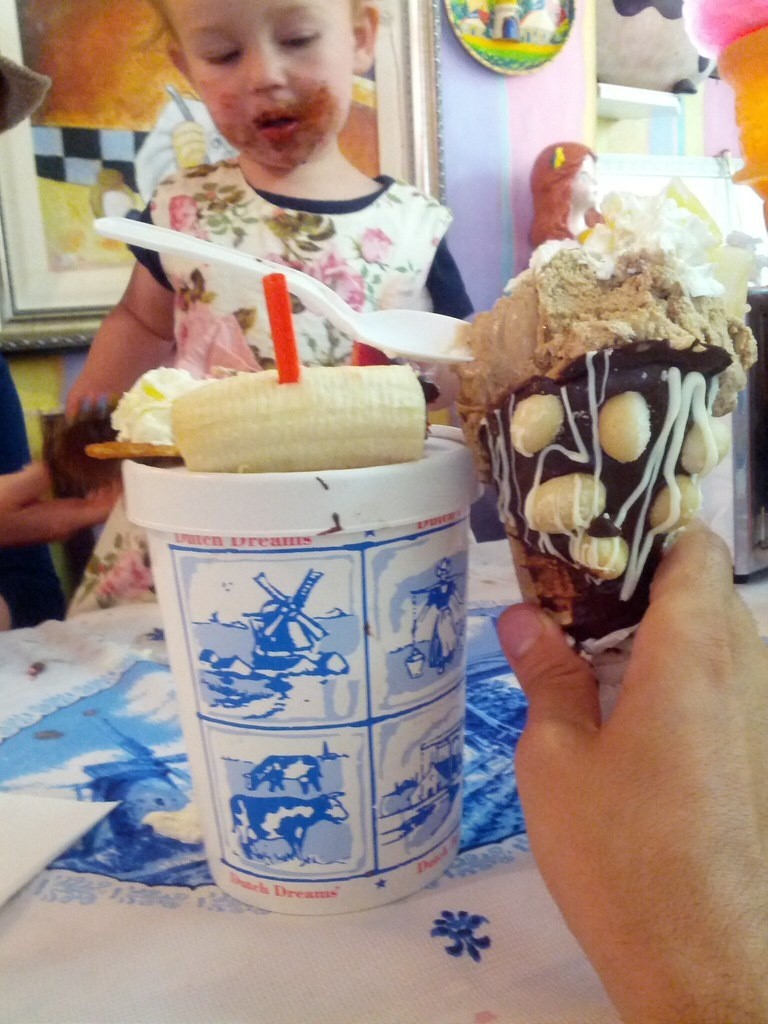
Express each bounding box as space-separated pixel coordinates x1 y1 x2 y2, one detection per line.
121 426 474 913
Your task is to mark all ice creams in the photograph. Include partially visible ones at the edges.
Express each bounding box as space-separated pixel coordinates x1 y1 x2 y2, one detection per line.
453 179 758 665
85 364 223 462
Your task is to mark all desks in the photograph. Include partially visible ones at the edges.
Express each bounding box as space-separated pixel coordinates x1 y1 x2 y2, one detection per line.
0 537 766 1024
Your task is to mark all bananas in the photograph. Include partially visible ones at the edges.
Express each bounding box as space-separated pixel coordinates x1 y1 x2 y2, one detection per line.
173 363 429 471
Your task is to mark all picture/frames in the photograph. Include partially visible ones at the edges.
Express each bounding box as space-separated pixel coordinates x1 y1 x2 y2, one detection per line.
2 1 447 349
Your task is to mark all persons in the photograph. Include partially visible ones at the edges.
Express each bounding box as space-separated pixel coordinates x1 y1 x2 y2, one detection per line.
0 52 129 632
65 2 472 620
497 521 768 1023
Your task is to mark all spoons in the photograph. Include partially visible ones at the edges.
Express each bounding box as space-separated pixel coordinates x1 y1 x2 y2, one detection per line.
94 214 478 365
